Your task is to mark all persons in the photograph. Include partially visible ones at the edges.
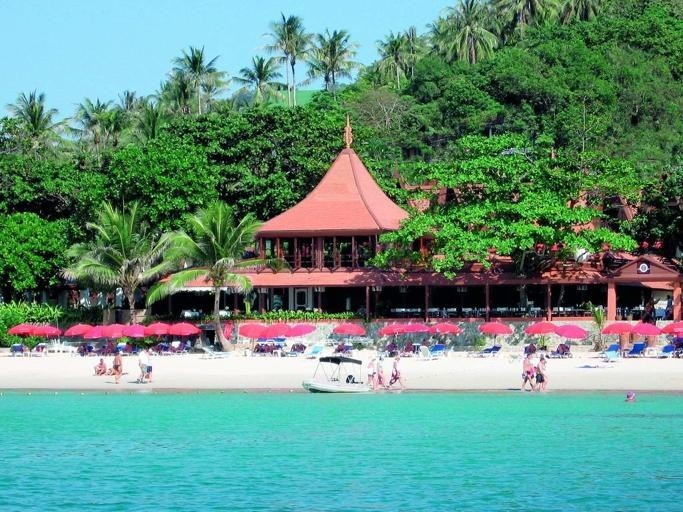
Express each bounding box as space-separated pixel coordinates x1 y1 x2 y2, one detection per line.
527 343 536 353
365 358 375 384
662 294 672 317
386 356 405 389
386 338 429 355
372 371 378 390
625 392 634 402
74 340 155 383
35 343 45 352
253 341 346 354
551 344 569 355
377 356 386 387
520 353 548 392
673 338 681 355
643 297 661 320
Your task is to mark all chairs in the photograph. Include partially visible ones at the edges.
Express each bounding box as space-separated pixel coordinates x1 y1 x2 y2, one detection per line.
9 337 683 360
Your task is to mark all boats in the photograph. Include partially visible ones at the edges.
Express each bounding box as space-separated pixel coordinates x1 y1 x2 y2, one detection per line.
301 357 372 393
